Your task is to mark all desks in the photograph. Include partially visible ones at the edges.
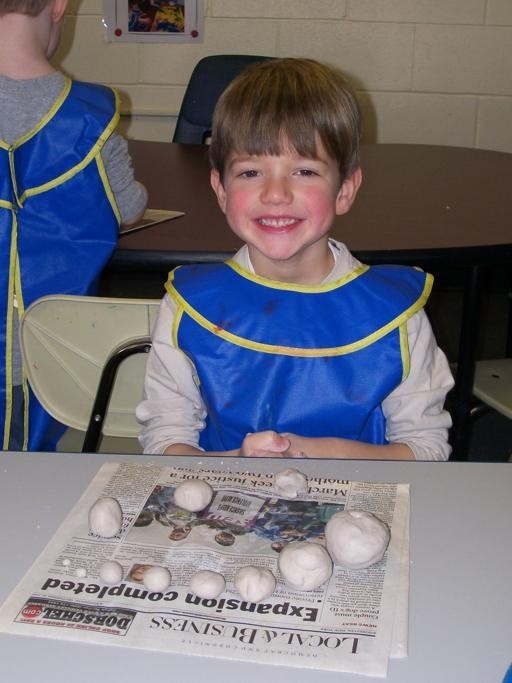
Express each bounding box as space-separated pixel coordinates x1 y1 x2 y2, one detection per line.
101 137 512 409
0 450 512 682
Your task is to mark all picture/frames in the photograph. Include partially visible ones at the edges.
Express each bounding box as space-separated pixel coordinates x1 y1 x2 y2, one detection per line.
102 0 205 43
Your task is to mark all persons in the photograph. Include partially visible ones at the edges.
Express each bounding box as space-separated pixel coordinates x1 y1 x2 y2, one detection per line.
128 565 154 583
0 1 148 451
133 56 456 462
167 489 215 541
135 485 168 527
254 500 327 553
202 492 269 546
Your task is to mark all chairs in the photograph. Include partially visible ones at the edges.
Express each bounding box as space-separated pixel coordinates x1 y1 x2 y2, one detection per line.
465 330 509 436
18 291 230 456
174 53 303 144
